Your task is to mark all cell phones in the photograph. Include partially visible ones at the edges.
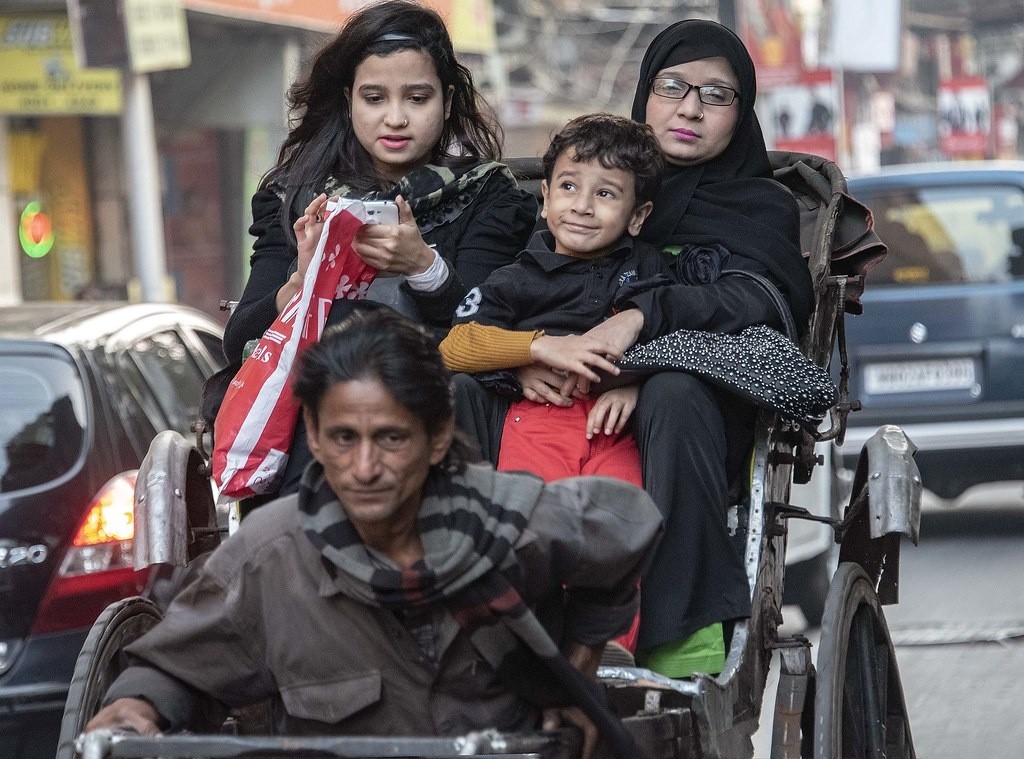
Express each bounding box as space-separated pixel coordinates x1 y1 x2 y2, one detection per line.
362 200 400 227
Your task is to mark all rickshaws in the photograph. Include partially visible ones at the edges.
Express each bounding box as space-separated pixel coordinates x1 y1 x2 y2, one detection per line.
58 161 921 759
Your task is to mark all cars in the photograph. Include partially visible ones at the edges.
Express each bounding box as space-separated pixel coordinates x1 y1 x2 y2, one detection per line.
2 305 229 719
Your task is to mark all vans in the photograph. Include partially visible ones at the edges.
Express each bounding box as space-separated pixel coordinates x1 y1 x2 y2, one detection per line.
832 160 1024 541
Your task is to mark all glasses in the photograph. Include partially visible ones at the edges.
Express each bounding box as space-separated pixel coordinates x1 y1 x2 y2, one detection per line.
649 77 742 106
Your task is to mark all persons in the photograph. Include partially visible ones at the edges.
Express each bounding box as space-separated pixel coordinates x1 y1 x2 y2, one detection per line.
89 308 663 757
445 21 816 681
201 1 537 469
439 114 671 667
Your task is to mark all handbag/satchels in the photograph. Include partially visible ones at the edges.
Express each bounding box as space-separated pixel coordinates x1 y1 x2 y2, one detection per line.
581 267 842 441
